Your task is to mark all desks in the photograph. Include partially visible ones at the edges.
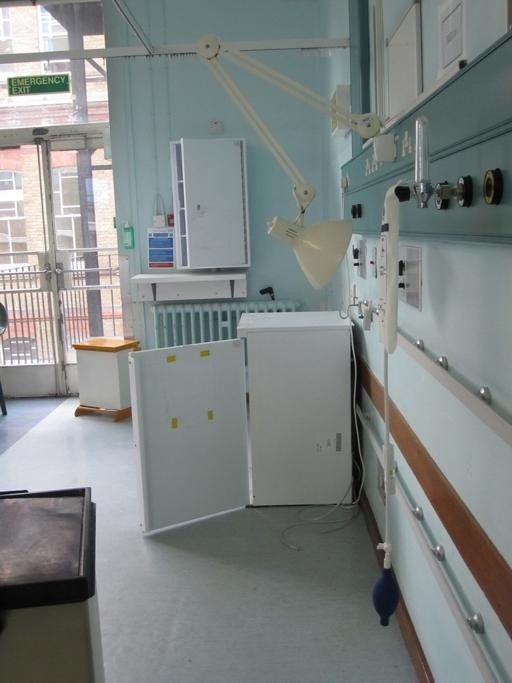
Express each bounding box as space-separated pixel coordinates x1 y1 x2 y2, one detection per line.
127 311 355 533
0 487 105 683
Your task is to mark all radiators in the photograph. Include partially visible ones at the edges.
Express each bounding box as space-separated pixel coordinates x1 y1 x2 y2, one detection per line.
151 299 300 370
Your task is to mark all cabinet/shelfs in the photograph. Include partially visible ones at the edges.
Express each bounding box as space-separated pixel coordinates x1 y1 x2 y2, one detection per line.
170 138 250 270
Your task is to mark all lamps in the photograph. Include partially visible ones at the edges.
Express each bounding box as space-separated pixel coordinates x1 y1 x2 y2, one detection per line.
197 36 395 291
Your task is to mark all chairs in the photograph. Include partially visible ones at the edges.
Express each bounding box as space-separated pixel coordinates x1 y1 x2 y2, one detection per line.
1 301 9 416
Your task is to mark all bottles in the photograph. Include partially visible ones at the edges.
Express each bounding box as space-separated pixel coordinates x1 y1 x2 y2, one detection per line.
155 193 166 228
415 115 430 184
166 195 174 227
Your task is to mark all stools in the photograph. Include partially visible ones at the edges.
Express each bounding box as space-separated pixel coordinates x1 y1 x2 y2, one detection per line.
73 338 139 422
